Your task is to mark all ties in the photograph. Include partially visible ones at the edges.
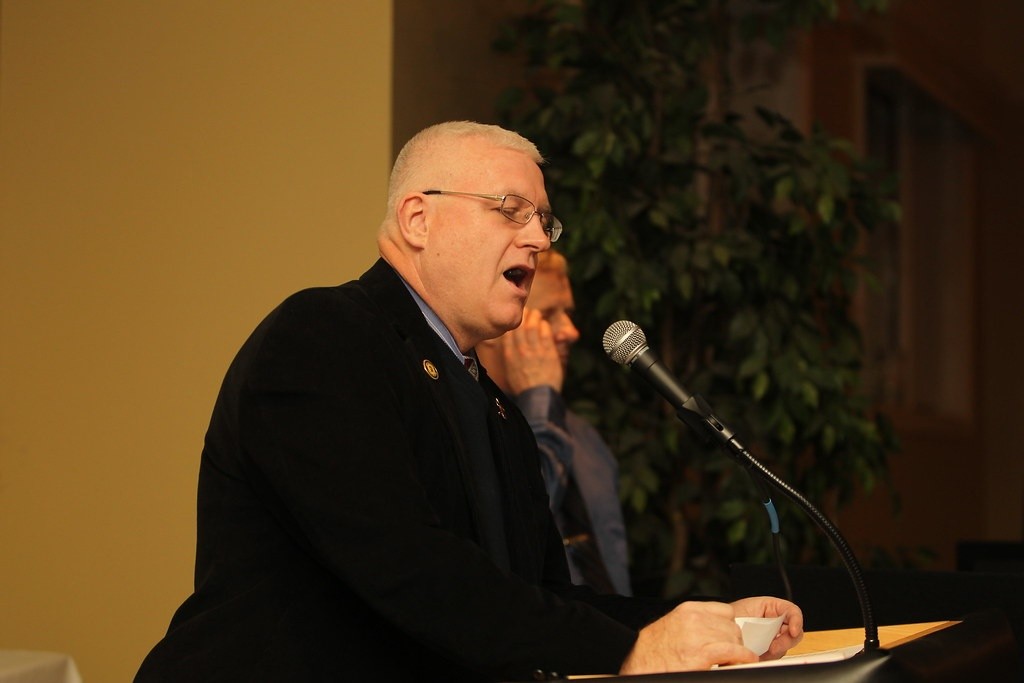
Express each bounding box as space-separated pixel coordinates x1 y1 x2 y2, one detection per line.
534 427 615 600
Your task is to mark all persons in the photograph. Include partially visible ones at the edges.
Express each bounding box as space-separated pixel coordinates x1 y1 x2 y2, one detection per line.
476 250 632 596
133 121 804 683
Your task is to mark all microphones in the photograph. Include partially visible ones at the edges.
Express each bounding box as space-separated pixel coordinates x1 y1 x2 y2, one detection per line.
601 320 744 455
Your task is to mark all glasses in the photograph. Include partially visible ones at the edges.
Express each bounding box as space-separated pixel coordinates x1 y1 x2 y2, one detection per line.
420 189 563 243
